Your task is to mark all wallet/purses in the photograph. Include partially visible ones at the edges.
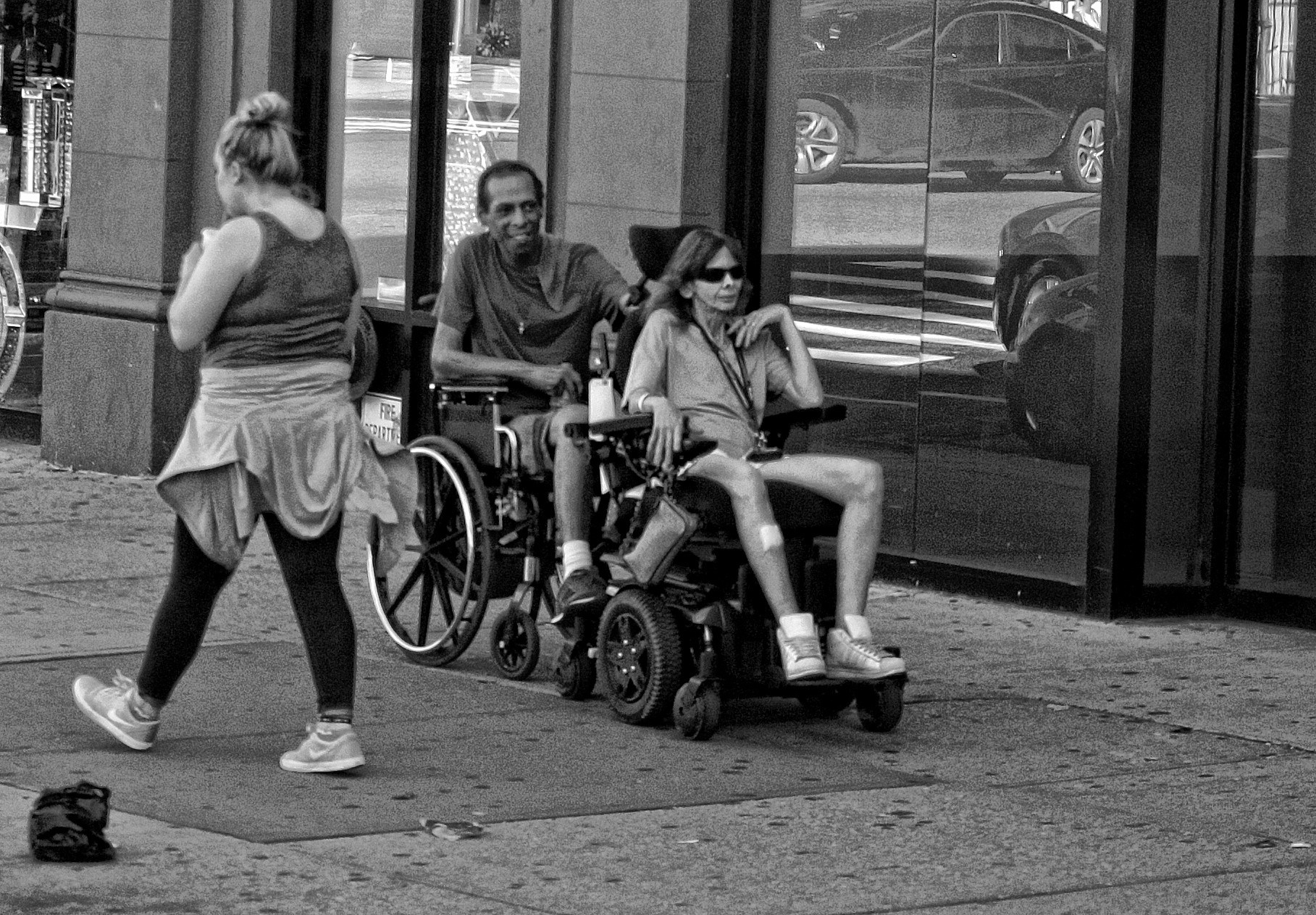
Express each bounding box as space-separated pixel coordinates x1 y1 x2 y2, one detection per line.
625 491 698 587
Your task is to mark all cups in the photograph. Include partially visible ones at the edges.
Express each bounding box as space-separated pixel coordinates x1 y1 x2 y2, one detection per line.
201 227 218 250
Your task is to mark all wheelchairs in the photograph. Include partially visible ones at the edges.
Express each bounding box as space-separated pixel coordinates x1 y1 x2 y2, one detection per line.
359 293 908 742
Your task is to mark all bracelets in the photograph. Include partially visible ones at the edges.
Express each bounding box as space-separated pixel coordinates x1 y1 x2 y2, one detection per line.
639 390 654 414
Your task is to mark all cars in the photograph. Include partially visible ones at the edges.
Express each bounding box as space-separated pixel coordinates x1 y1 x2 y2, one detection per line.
987 143 1316 493
792 0 1109 196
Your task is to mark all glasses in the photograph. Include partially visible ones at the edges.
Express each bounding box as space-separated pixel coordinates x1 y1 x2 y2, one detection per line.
697 264 744 283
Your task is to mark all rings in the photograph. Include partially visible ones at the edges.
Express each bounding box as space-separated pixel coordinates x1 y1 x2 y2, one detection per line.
752 323 757 327
742 317 746 325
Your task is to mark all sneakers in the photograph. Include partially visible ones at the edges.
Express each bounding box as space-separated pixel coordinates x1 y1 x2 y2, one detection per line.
777 612 827 682
72 675 161 751
556 569 608 613
280 724 365 773
825 613 906 679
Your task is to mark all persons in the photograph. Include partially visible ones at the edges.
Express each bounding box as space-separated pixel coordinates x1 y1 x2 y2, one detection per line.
623 229 907 683
70 92 419 773
430 160 641 627
